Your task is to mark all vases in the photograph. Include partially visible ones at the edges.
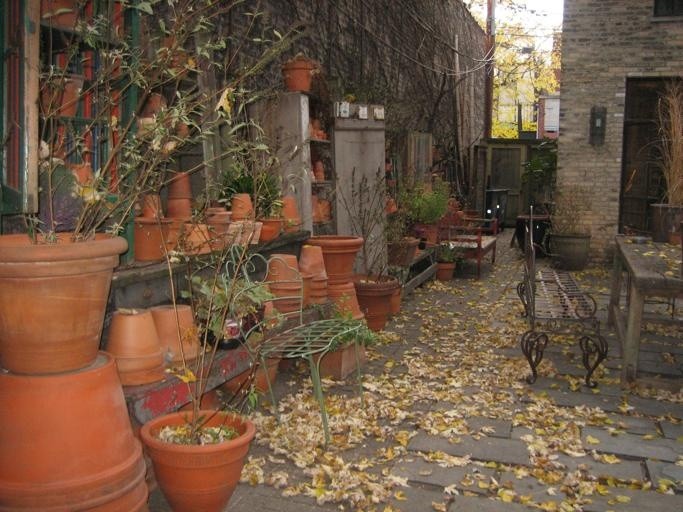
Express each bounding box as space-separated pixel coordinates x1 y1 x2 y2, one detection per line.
644 202 683 245
1 2 438 512
665 228 681 243
548 232 592 272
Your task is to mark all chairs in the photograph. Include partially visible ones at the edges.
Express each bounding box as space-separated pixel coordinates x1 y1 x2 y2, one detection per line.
439 201 499 283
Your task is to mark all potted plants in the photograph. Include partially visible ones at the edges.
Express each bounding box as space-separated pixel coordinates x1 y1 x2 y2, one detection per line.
432 247 457 281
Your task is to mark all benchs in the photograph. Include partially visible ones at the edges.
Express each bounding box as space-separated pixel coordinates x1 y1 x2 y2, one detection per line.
512 201 612 399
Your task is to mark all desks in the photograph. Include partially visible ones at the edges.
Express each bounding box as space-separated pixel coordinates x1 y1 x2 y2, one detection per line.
608 231 683 394
